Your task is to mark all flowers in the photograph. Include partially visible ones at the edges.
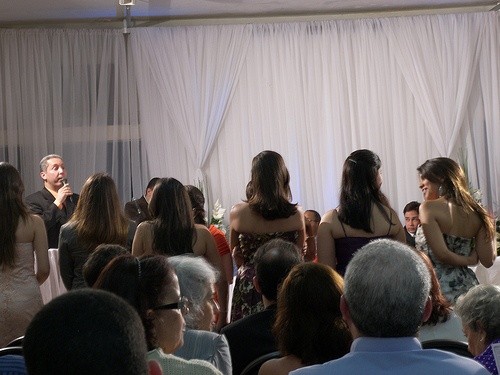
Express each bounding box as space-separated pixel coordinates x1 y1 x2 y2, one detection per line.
210 197 227 235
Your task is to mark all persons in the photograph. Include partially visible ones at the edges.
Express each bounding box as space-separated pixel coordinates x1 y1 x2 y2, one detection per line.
0 162 50 348
220 239 302 375
59 174 136 290
305 209 321 253
456 283 500 375
23 288 164 375
26 157 79 248
83 244 129 287
260 265 353 375
317 153 406 277
186 184 234 324
95 254 223 375
131 177 228 330
417 158 496 305
168 256 233 375
230 150 307 322
125 178 159 224
290 240 490 375
414 251 466 343
404 202 422 238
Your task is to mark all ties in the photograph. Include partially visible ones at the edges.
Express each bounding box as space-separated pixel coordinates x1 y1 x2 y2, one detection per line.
62 203 67 215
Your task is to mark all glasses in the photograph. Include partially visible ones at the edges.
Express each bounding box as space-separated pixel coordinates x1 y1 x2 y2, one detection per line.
152 296 190 316
305 218 318 223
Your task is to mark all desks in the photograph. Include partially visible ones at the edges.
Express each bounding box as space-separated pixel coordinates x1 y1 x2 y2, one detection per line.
33 248 69 306
466 256 500 286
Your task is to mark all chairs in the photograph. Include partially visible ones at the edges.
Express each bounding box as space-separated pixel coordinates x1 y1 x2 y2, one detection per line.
4 335 25 348
239 350 285 375
417 305 469 343
0 346 25 356
421 339 477 359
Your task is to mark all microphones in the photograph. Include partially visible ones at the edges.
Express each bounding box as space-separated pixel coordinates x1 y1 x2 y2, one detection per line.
61 178 74 202
